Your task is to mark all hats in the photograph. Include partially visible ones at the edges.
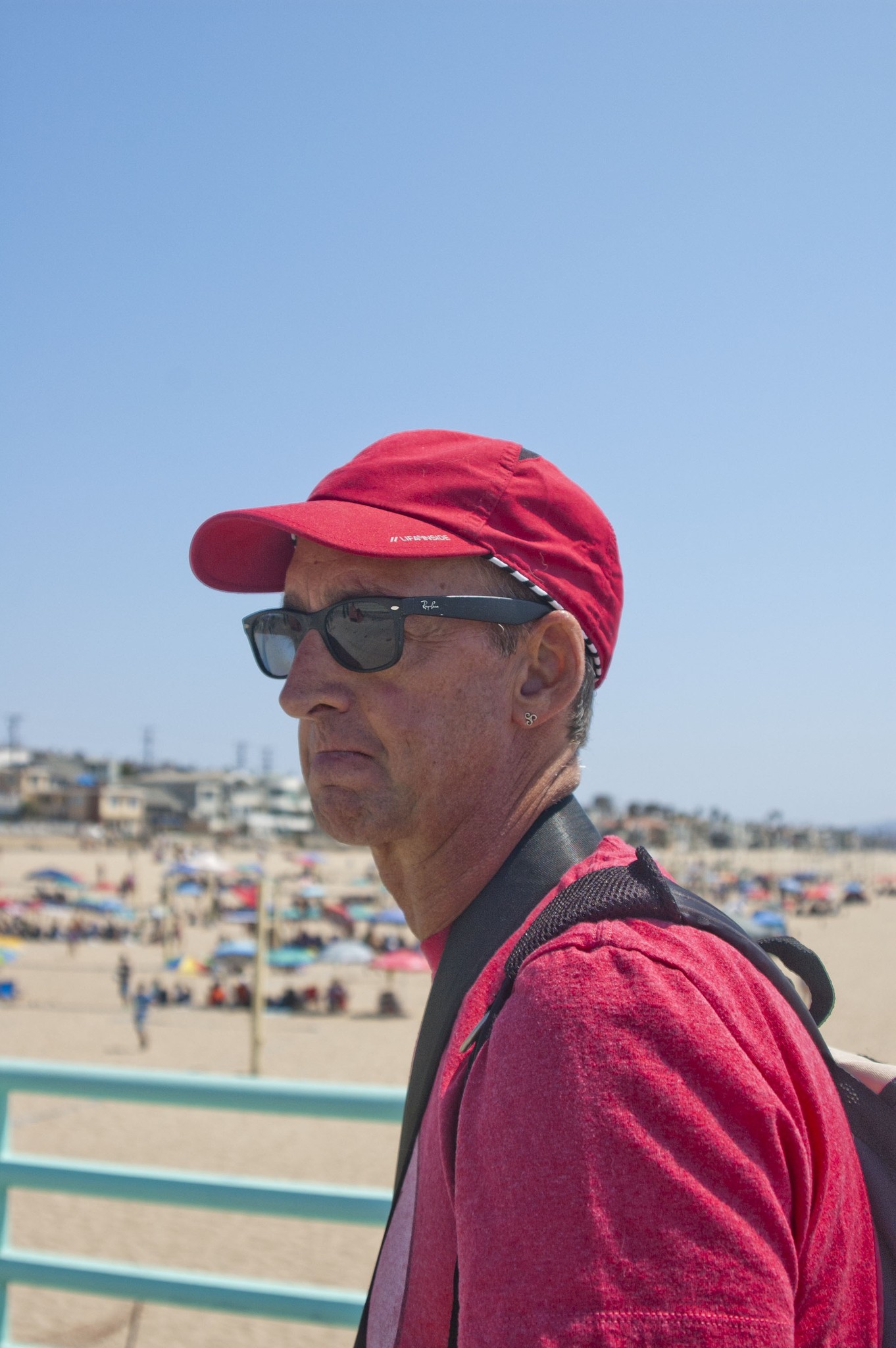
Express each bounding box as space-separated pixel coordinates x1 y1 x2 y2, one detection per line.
189 429 625 690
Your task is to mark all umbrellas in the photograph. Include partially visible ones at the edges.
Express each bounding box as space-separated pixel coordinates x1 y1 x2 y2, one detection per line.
25 860 864 970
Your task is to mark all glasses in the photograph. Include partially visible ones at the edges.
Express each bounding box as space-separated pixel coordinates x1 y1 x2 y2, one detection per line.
241 594 553 679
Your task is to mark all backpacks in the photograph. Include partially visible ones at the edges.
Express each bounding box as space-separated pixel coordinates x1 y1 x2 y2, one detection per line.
447 845 896 1348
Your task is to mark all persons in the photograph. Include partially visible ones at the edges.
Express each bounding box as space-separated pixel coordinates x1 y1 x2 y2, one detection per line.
13 845 409 1048
190 431 881 1348
